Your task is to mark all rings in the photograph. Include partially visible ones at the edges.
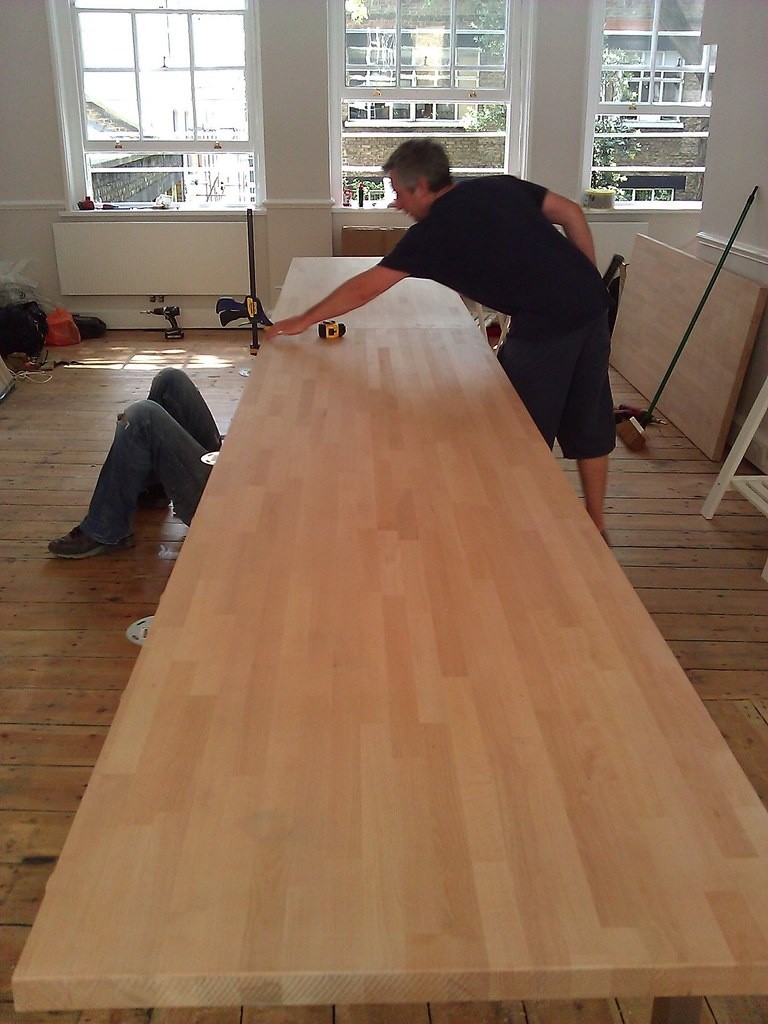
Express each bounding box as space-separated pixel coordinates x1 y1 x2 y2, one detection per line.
278 330 282 333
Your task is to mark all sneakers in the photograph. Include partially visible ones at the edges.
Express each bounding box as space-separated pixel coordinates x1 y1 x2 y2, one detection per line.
136 493 171 509
48 526 136 558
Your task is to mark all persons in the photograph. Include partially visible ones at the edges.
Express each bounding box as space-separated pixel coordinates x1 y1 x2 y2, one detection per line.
47 366 222 559
266 140 616 535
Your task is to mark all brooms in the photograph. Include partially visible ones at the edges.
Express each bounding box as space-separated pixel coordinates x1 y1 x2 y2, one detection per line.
615 184 759 453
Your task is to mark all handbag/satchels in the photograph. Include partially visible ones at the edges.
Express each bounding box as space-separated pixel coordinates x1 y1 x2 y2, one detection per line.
45 308 81 346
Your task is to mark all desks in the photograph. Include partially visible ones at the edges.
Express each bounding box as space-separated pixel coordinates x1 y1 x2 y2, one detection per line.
13 257 768 1024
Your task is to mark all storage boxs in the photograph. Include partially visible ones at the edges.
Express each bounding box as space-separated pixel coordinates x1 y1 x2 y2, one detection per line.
342 226 410 257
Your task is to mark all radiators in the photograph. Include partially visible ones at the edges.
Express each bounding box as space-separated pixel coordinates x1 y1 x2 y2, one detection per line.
52 221 252 296
